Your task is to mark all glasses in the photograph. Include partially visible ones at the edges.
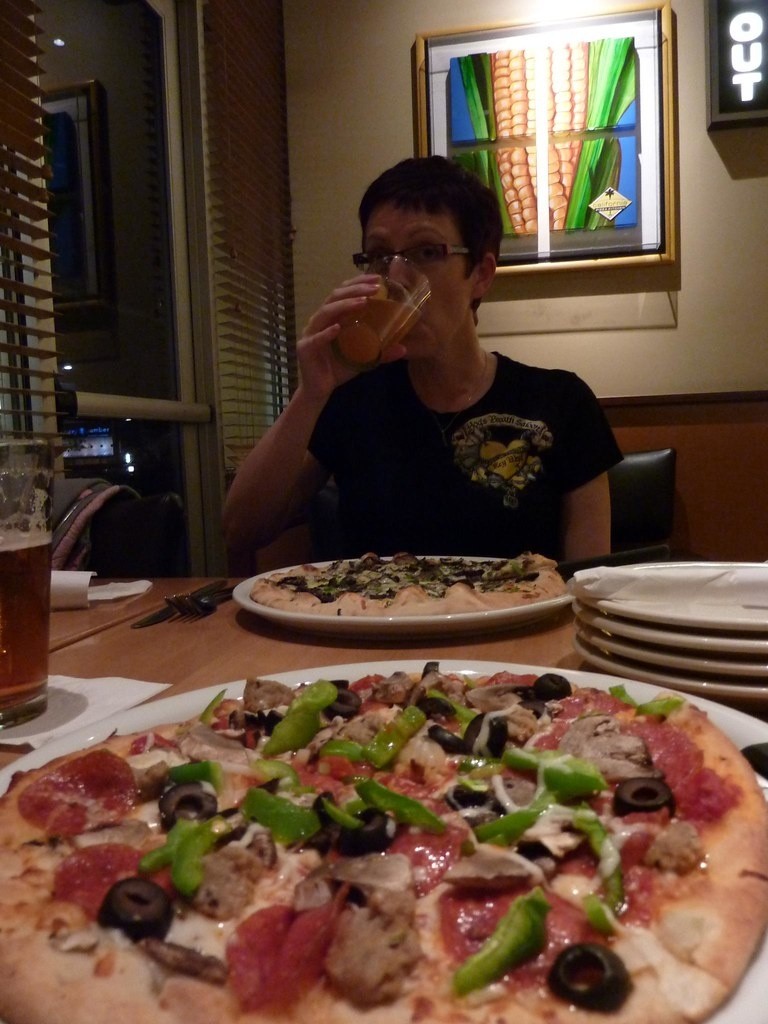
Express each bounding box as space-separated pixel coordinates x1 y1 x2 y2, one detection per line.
352 243 471 275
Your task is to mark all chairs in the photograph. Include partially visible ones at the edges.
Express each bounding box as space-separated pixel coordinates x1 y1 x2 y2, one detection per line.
608 448 682 548
87 492 193 578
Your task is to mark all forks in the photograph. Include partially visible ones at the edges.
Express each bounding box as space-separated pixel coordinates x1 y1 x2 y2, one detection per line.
163 583 237 617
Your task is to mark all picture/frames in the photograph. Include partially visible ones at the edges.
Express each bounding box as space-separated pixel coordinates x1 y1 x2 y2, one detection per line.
413 0 682 275
40 75 121 317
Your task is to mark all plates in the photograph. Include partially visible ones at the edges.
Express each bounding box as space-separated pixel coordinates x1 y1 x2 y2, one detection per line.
0 655 768 1024
567 560 768 712
232 554 576 635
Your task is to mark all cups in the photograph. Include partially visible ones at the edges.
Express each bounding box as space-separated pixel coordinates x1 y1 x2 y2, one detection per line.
0 431 56 733
330 254 431 371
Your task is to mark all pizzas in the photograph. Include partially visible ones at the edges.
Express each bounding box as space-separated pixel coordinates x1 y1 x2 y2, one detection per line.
0 660 768 1024
250 551 567 617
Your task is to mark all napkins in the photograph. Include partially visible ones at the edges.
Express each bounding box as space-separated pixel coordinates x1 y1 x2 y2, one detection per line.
0 674 173 751
87 580 153 602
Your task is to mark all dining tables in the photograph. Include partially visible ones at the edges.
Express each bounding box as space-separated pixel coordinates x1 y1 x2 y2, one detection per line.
0 573 606 771
47 577 223 657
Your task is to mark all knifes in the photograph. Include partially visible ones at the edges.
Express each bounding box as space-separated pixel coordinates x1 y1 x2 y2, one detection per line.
130 581 227 629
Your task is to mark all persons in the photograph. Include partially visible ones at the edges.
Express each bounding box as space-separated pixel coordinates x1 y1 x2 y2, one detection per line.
214 157 626 564
